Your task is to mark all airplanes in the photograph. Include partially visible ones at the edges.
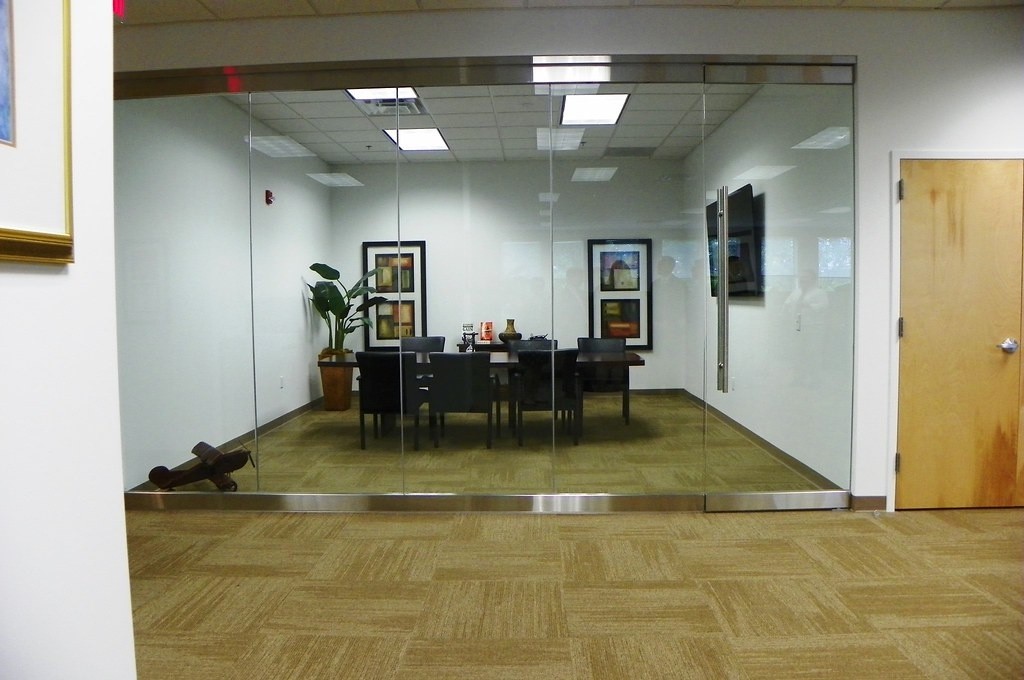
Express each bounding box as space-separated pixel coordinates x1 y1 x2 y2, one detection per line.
149 442 256 492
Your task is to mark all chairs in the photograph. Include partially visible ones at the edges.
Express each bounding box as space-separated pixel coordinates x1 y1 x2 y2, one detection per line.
577 337 627 419
506 339 558 429
355 352 432 451
400 336 445 437
517 351 580 447
429 352 502 450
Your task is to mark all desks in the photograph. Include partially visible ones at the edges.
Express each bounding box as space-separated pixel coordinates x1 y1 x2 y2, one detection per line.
457 340 511 352
317 352 645 438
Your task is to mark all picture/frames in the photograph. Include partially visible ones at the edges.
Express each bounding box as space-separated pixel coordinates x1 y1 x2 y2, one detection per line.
363 241 427 351
0 0 76 265
587 238 653 349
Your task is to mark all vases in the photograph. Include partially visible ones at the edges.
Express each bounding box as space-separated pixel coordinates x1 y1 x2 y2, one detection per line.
499 319 522 344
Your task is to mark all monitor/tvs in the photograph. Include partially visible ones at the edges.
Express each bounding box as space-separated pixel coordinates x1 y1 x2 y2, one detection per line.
706 184 761 298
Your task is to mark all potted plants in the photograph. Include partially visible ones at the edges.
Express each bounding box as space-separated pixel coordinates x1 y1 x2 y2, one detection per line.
307 263 388 411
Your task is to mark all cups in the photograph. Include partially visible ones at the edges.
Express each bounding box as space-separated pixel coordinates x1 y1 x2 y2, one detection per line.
463 324 473 339
